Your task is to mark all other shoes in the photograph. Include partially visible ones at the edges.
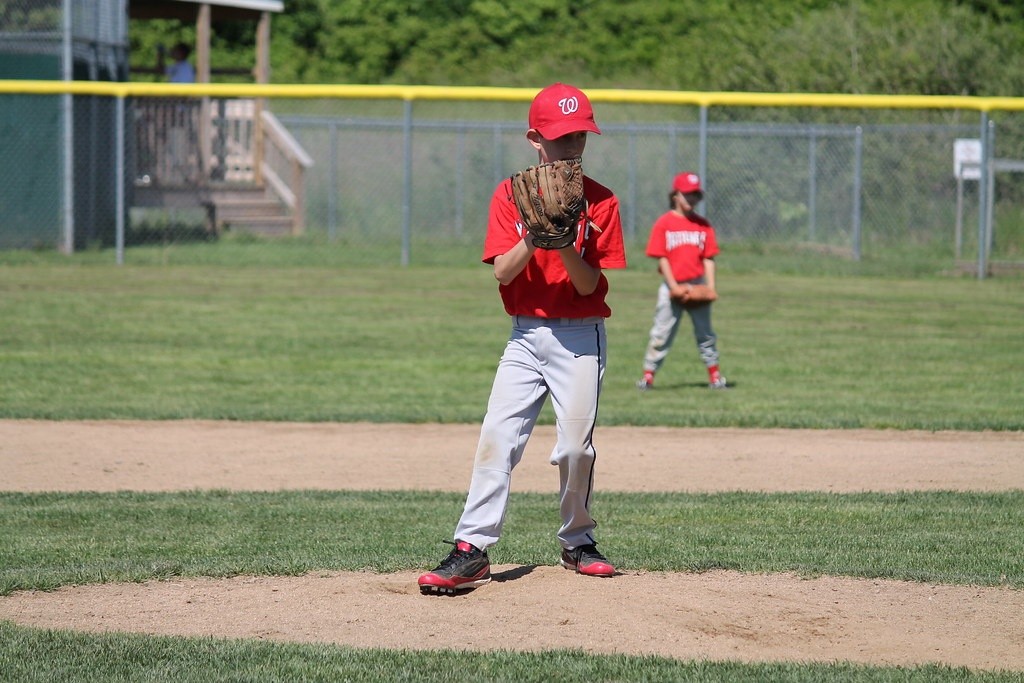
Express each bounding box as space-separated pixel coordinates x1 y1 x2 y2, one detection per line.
635 380 653 389
708 378 727 391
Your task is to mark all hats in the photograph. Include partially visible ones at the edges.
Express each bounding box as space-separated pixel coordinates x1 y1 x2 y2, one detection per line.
674 172 704 194
528 82 601 140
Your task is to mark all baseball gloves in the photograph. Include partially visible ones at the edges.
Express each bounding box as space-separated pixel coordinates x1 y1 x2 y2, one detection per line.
510 157 585 251
668 286 716 301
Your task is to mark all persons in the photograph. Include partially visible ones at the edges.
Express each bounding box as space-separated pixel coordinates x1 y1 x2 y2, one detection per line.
417 83 628 594
636 172 727 390
166 39 195 84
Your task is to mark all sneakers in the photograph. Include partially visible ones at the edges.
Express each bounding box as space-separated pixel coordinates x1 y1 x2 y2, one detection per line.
417 540 491 596
560 543 616 576
